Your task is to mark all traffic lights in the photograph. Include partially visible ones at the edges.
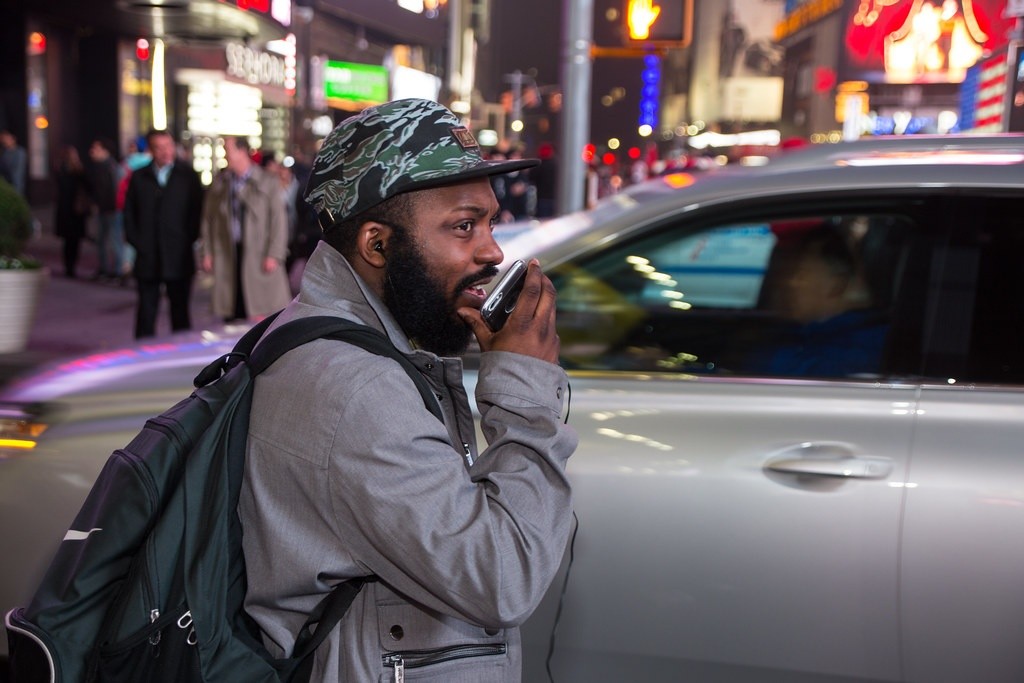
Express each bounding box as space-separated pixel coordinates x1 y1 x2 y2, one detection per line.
622 0 694 48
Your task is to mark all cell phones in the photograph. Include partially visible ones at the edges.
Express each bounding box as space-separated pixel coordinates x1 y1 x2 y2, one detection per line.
481 260 529 332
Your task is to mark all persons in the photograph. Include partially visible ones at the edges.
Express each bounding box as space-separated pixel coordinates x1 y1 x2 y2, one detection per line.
69 135 123 285
200 135 292 325
0 124 26 196
123 130 205 340
257 148 556 300
240 97 578 683
53 145 92 278
628 222 887 381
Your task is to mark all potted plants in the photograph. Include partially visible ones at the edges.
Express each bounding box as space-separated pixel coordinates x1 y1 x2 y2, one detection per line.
0 176 53 355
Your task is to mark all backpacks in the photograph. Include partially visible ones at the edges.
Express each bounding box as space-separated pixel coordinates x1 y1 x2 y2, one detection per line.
0 307 445 683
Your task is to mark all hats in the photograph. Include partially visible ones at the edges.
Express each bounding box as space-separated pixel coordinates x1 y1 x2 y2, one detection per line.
772 215 892 273
303 98 544 232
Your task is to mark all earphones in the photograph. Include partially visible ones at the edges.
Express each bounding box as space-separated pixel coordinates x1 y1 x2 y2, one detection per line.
374 241 383 251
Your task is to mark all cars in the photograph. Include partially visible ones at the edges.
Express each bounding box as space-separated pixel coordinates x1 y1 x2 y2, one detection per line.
0 132 1024 683
